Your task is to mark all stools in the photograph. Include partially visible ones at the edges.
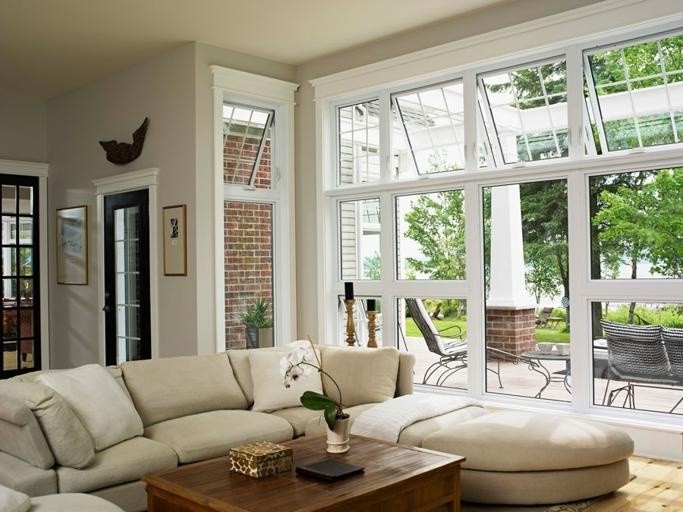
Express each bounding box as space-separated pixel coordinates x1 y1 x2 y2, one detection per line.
305 393 488 447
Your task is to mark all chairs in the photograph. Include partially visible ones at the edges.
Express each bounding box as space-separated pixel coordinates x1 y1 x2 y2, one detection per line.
357 298 415 375
405 295 538 389
0 482 125 512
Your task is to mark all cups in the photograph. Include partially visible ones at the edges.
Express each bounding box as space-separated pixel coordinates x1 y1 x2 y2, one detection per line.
538 343 570 355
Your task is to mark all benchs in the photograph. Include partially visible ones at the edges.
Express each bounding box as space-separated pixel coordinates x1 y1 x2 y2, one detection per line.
597 336 683 413
420 407 636 506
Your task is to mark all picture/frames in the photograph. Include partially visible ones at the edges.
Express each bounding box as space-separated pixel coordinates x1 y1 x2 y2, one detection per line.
162 204 188 276
56 205 88 285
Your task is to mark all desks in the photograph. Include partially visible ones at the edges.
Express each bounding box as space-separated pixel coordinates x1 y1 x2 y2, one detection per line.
521 349 572 400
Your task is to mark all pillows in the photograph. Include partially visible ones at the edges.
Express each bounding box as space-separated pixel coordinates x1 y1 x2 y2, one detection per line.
248 340 322 413
599 319 672 377
659 324 683 378
322 347 399 403
0 352 248 468
0 483 31 512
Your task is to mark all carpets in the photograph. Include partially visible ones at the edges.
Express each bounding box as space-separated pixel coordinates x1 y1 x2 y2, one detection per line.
456 470 636 512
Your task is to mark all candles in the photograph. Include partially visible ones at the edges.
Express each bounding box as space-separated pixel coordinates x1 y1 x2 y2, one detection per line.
345 282 353 299
367 299 375 311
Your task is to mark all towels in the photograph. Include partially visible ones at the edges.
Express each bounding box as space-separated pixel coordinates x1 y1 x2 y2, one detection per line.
351 392 485 443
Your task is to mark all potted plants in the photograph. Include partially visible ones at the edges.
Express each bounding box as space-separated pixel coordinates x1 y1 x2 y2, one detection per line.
298 391 351 455
239 296 272 348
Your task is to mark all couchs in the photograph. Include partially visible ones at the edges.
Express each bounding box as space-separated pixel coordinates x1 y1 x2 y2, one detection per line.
0 353 416 512
